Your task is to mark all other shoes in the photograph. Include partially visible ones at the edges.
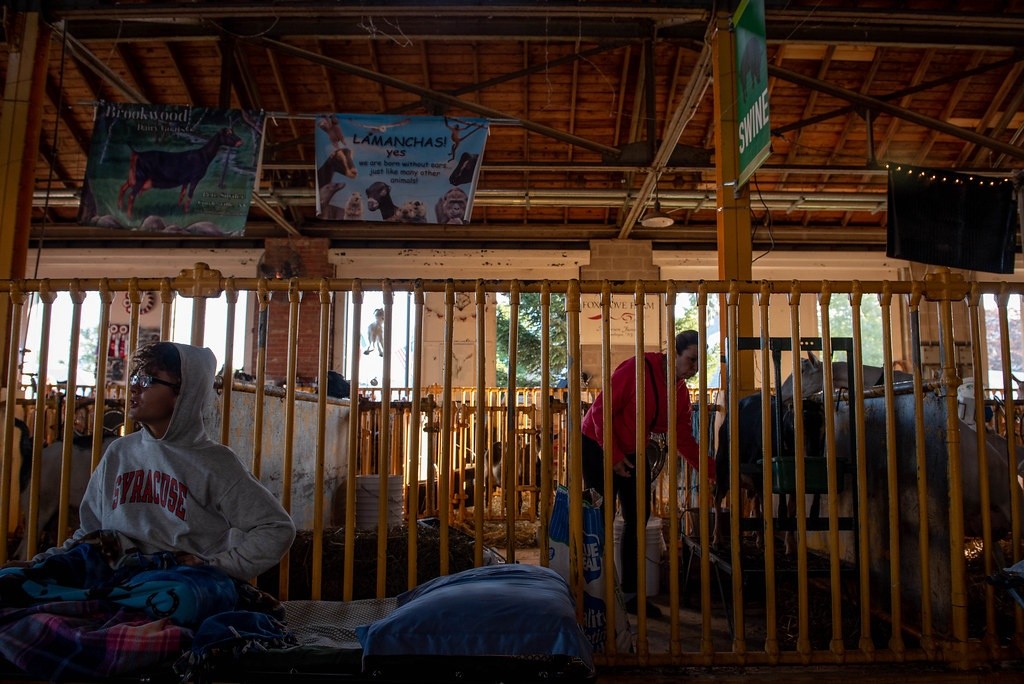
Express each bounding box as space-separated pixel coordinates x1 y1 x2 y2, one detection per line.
625 597 662 620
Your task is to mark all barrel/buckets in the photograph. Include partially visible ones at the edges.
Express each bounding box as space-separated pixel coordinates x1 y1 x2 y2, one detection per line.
613 518 668 595
355 475 404 529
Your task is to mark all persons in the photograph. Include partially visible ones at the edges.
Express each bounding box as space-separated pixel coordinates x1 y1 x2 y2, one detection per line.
0 341 296 683
582 331 717 618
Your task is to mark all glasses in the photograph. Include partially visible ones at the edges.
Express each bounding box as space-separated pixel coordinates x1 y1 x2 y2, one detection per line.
129 375 170 388
